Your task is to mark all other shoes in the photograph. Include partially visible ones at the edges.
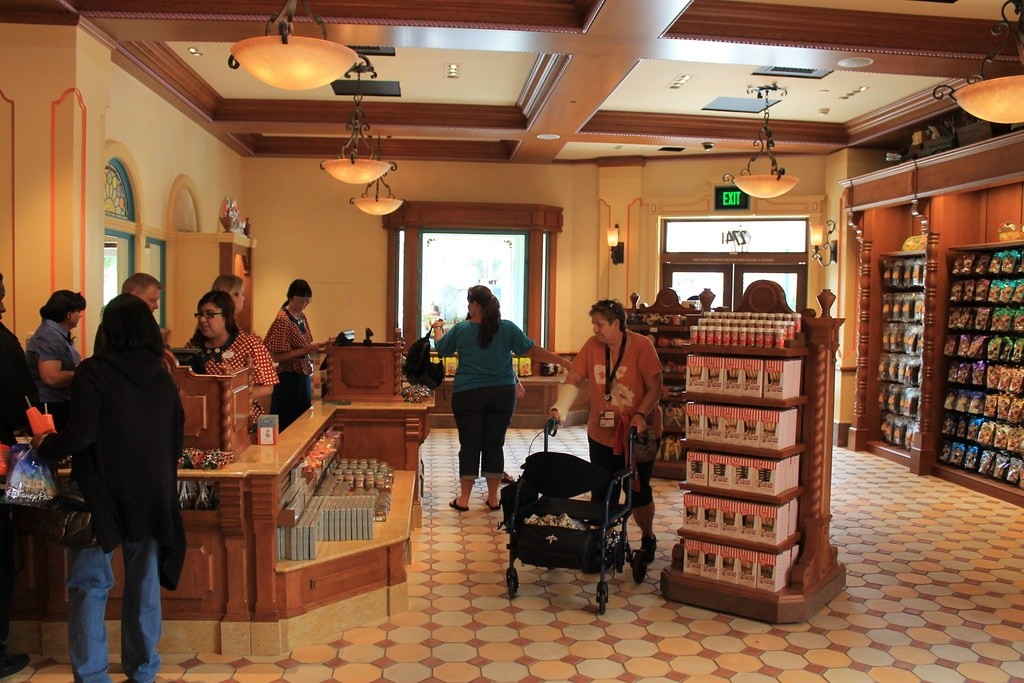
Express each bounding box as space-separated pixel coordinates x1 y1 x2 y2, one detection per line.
641 534 656 563
502 472 513 483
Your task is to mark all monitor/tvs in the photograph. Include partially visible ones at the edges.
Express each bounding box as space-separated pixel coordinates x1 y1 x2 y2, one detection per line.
319 330 355 370
170 348 206 375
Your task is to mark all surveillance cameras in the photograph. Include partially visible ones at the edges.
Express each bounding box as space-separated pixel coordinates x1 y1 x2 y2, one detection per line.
703 143 714 151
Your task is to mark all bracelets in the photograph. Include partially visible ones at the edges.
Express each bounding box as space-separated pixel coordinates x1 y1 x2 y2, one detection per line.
434 326 441 332
634 412 646 419
563 358 566 366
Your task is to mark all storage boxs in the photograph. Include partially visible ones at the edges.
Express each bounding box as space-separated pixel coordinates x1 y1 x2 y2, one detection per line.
277 462 376 561
258 414 278 445
684 354 802 593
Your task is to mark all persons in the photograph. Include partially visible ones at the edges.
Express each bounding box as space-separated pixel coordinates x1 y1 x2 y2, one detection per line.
433 285 574 512
264 279 332 434
43 294 188 682
547 299 663 564
0 273 279 683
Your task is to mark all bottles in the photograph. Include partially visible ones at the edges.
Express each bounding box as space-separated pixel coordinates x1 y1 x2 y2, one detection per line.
690 312 801 349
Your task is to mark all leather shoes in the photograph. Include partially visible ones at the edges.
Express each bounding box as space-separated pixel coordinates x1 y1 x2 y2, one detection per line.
0 653 30 679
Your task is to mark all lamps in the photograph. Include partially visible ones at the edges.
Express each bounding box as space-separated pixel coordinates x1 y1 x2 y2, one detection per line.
606 223 624 265
228 0 406 215
933 0 1024 125
722 84 800 199
809 219 837 267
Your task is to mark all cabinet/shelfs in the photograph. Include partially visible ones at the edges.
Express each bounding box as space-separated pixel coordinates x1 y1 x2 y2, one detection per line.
660 280 846 623
624 288 730 480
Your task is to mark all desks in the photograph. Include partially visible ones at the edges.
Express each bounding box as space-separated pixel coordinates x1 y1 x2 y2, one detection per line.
429 373 589 428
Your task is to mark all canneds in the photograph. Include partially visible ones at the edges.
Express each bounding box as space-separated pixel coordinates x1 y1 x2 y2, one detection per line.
673 314 681 325
690 312 801 350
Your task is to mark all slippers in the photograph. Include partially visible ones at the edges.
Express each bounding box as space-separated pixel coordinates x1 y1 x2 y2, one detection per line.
486 498 502 510
449 497 469 511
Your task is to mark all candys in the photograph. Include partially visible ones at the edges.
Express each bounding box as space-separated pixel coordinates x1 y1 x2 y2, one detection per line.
177 447 227 471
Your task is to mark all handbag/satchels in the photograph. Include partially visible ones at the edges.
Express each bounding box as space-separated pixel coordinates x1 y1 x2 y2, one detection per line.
623 418 657 462
404 325 446 389
0 440 94 548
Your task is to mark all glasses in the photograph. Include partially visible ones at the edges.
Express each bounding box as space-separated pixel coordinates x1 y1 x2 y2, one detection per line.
598 301 621 315
293 297 312 303
194 312 222 320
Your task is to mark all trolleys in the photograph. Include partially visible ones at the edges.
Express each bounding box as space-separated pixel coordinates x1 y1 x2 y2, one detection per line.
495 418 647 617
